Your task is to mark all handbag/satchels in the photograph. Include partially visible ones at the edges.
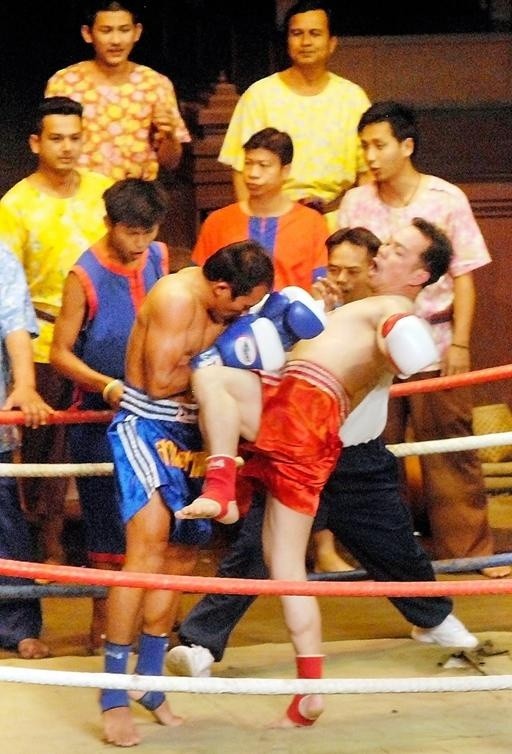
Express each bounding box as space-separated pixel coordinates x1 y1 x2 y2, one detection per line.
451 342 473 352
101 379 123 402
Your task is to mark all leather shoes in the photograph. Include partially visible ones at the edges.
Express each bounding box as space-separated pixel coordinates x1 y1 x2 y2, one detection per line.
250 286 326 351
190 318 285 374
380 312 442 379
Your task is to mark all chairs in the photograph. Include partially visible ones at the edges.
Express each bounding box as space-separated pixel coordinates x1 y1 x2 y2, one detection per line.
410 614 479 649
167 645 212 680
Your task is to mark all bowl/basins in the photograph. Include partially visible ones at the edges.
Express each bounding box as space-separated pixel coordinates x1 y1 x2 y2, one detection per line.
379 170 421 208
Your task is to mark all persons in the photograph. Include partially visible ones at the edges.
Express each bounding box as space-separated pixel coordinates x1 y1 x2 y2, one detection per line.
0 93 121 591
157 224 482 684
323 97 512 580
96 239 325 750
216 1 376 241
0 237 59 662
181 125 335 316
45 177 173 665
40 2 194 183
170 215 455 731
